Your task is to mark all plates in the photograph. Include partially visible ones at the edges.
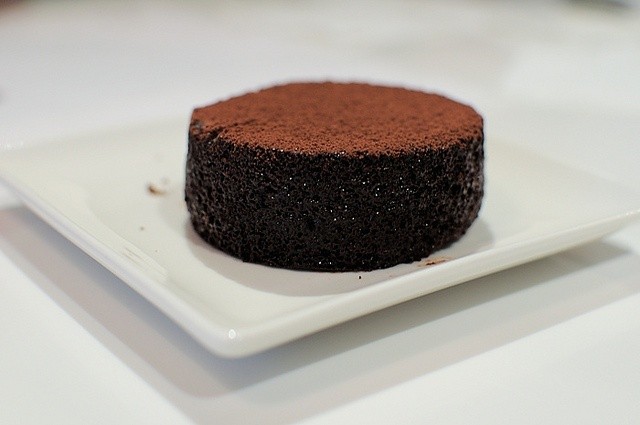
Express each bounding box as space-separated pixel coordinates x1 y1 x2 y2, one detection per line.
1 106 640 364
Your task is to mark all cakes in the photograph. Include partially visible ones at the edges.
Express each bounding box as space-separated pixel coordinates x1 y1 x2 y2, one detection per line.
185 83 488 273
